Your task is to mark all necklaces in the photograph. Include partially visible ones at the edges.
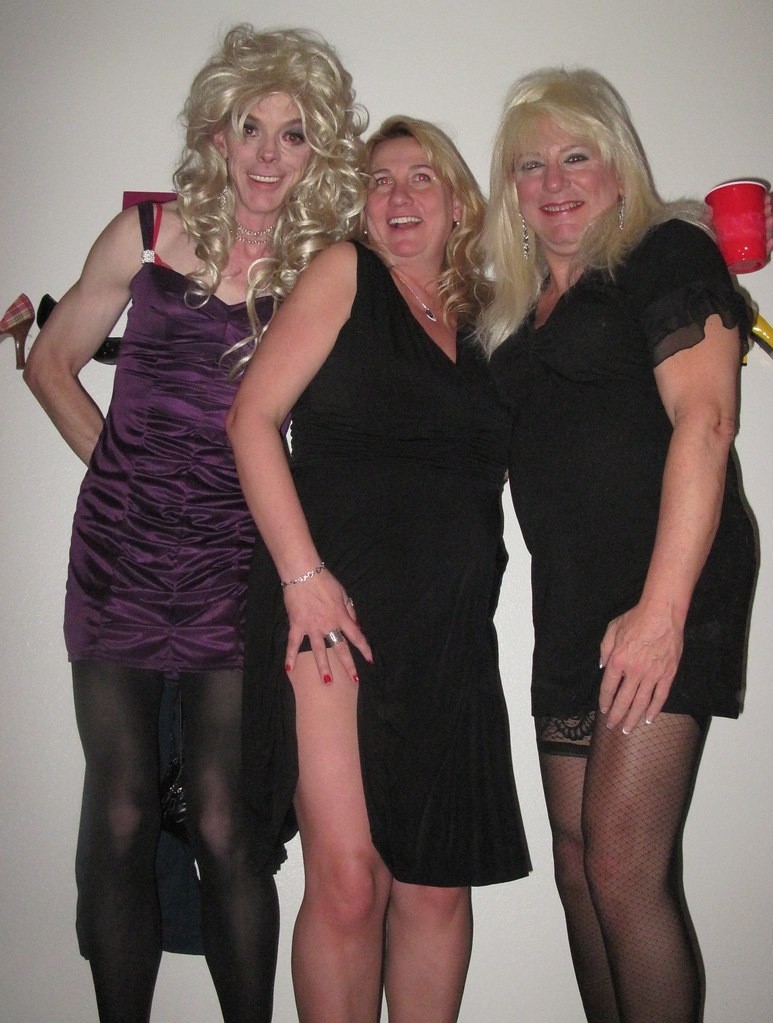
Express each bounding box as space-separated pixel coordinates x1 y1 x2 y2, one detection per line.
399 277 438 322
235 215 275 244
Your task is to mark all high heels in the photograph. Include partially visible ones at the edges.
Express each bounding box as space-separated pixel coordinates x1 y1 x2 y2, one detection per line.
0 292 35 370
36 294 123 365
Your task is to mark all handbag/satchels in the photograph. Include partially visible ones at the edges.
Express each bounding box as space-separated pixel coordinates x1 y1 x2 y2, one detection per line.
152 690 219 955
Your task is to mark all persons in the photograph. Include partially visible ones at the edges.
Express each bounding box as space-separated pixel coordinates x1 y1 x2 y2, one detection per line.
24 26 370 1023
226 114 773 1023
492 71 761 1023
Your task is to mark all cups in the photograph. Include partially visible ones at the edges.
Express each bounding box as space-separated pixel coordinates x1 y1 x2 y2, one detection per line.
704 181 767 275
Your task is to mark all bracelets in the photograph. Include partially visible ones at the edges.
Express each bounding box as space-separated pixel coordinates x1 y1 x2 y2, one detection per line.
282 562 326 588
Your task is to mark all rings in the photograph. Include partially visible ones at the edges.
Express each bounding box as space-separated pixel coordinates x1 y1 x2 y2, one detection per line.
345 596 354 607
326 630 345 646
